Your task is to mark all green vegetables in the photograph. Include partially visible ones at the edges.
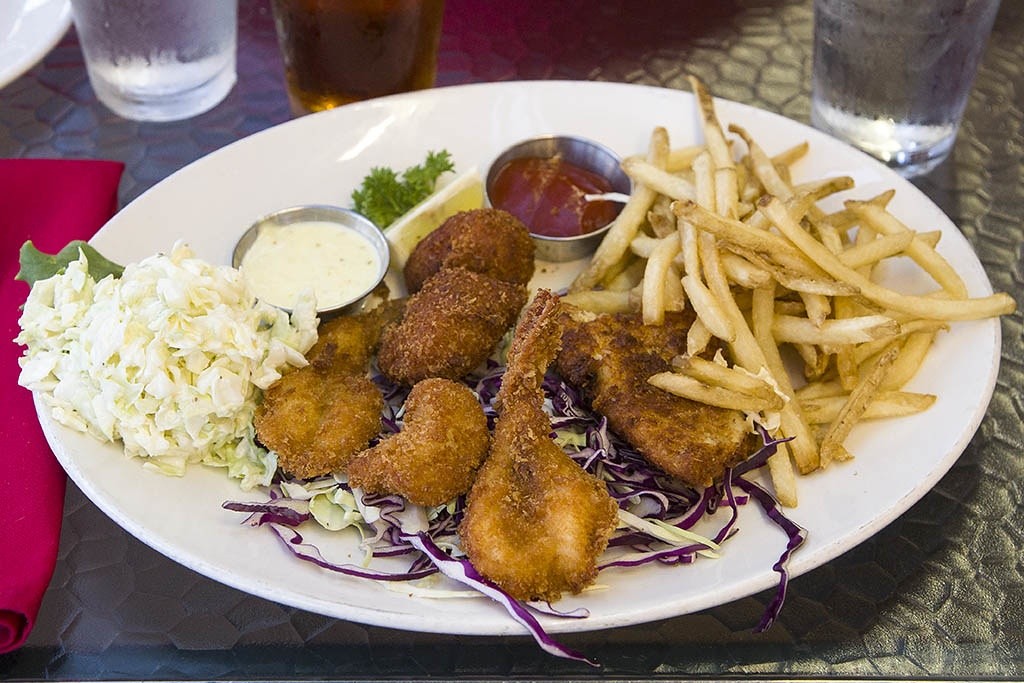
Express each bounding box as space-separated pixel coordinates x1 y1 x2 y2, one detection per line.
353 149 456 228
16 239 128 311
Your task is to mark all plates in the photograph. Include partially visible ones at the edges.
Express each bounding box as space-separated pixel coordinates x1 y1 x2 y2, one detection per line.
33 81 1001 636
0 0 73 91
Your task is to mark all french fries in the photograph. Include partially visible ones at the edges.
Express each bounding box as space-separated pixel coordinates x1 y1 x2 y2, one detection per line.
554 74 1017 509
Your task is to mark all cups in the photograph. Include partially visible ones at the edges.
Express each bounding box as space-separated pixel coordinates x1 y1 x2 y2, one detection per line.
70 0 239 121
808 0 1000 179
272 0 445 118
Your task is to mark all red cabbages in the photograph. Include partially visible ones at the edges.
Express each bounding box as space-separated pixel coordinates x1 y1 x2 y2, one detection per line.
221 287 807 666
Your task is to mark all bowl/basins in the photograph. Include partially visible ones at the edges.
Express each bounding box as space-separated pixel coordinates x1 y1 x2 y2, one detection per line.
231 205 391 324
485 134 635 262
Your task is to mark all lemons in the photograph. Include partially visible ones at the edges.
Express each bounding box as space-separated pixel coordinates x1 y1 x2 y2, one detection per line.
382 173 484 272
